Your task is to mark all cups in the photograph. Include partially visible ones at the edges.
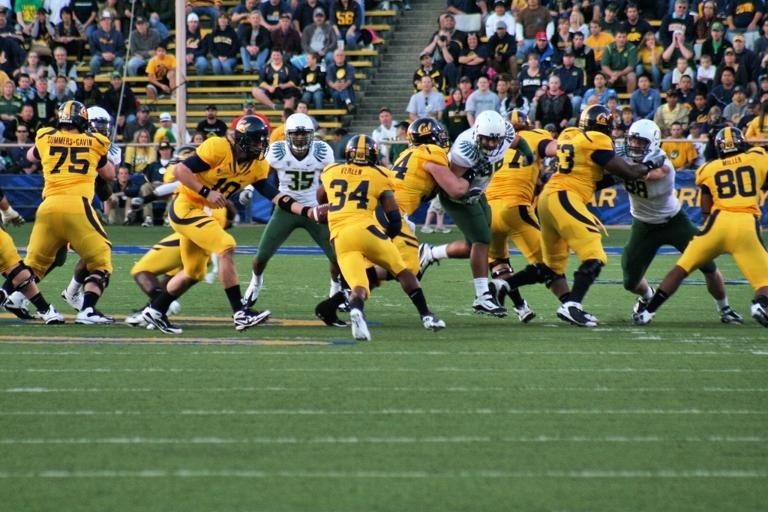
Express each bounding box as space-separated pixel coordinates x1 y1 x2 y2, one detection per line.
336 40 345 50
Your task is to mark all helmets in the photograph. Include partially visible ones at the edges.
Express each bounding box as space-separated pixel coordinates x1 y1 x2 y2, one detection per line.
506 112 534 132
87 105 114 124
235 113 268 145
57 99 87 127
283 112 316 140
344 134 378 166
627 118 662 156
578 105 613 135
715 126 746 156
472 110 506 143
407 116 443 145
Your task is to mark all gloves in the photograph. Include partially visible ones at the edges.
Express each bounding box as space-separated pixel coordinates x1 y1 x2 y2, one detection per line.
130 198 144 209
644 154 666 170
239 189 253 206
1 206 25 228
471 154 491 174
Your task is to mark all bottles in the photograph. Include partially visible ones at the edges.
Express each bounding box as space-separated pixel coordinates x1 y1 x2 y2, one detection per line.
321 58 326 69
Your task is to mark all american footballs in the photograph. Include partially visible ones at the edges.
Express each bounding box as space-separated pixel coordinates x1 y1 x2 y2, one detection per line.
309 203 330 224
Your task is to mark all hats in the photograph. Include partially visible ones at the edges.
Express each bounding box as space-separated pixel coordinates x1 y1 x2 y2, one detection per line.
156 141 174 154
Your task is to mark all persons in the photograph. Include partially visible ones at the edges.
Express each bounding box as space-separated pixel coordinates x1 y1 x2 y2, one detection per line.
0 0 411 228
315 118 475 326
597 119 743 324
320 134 446 341
371 0 768 234
141 115 330 334
0 100 122 324
488 105 665 327
244 113 350 312
417 110 535 316
634 126 768 327
127 147 226 327
485 125 569 321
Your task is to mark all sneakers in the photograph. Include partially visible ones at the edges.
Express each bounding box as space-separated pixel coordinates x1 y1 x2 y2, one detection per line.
0 289 66 325
751 296 768 328
419 225 454 236
232 277 272 331
124 299 184 335
61 289 85 312
630 286 657 325
97 212 170 227
421 313 447 331
471 278 537 324
314 268 371 341
74 308 116 324
415 242 437 283
719 305 744 325
556 301 599 327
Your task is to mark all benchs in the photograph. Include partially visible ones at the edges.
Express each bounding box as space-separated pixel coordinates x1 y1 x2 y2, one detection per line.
67 10 396 142
617 92 667 99
647 20 663 27
